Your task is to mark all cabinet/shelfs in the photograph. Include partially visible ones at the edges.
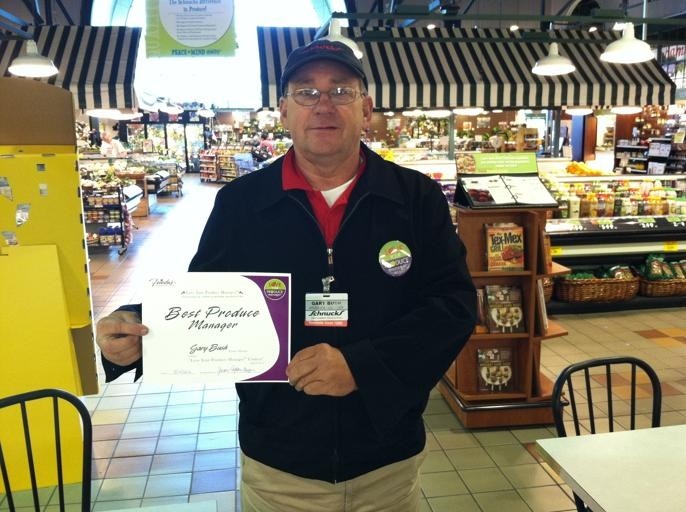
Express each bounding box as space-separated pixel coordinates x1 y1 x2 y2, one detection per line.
615 141 686 174
78 184 129 254
218 155 236 183
437 207 571 432
199 150 218 182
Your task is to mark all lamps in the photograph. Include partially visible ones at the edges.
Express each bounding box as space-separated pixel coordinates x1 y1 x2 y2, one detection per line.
8 27 59 80
317 10 686 83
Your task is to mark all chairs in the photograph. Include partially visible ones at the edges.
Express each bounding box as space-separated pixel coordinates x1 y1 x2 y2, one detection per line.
551 356 662 512
0 390 93 512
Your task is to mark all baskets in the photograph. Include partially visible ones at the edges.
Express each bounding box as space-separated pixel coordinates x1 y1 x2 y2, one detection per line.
543 282 553 303
556 276 641 304
640 279 686 297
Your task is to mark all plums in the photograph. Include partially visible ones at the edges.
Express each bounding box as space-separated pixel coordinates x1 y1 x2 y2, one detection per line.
468 190 488 202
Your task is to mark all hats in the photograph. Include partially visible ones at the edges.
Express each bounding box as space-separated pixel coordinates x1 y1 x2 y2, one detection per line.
280 39 369 93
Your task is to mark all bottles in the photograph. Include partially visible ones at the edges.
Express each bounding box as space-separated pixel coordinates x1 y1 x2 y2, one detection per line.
541 180 686 220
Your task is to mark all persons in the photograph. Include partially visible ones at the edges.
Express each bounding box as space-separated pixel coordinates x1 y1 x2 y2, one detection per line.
94 39 478 512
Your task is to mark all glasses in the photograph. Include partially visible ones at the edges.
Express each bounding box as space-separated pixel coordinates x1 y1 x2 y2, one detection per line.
285 86 364 106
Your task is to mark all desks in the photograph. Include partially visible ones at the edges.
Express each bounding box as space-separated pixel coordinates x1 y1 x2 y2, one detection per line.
536 423 686 512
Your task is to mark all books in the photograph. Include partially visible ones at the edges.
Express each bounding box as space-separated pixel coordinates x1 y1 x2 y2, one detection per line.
458 174 559 209
474 220 554 397
